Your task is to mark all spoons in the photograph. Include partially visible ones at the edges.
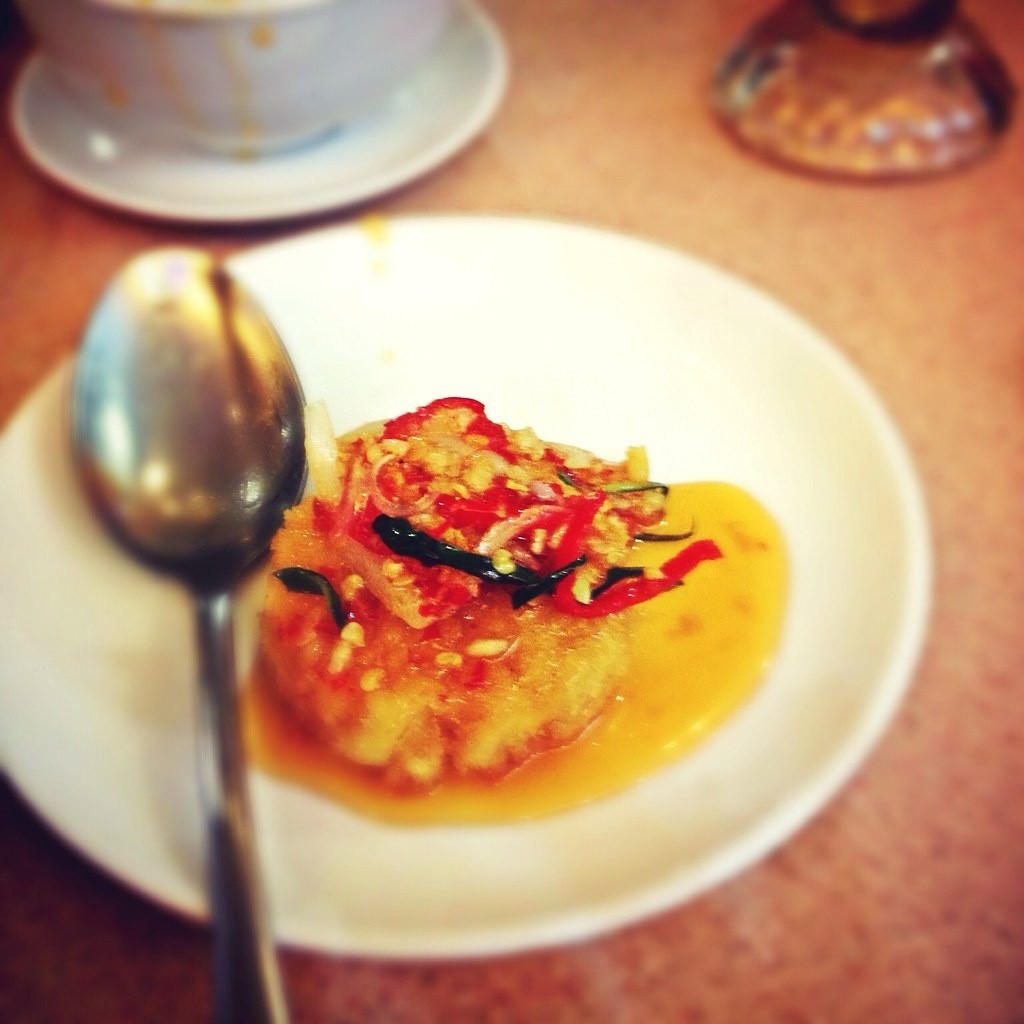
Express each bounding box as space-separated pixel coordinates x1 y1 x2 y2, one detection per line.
71 246 309 1024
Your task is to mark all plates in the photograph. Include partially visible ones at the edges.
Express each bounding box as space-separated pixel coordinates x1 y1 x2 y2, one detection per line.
12 0 508 222
0 215 933 958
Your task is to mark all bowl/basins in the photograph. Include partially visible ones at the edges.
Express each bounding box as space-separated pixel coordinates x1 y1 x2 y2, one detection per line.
18 0 452 151
